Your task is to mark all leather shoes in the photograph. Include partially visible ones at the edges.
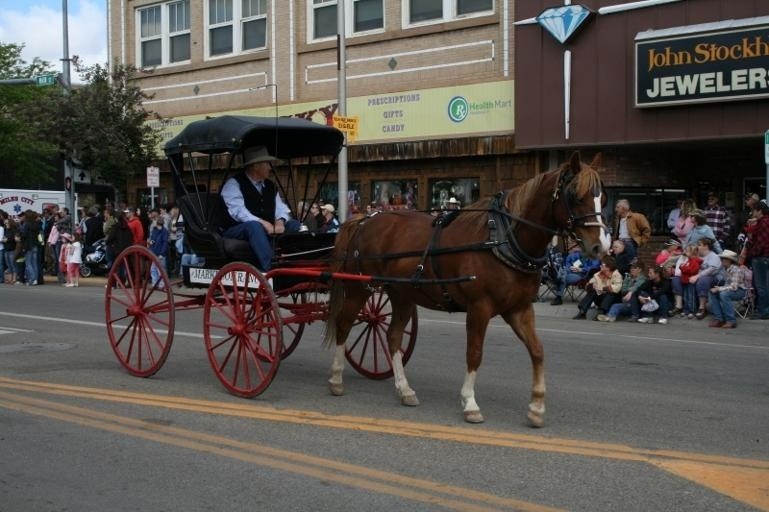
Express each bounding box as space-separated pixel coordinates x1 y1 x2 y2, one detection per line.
709 320 736 328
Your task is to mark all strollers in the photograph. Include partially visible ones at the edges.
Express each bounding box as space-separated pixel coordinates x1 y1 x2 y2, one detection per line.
79 237 114 278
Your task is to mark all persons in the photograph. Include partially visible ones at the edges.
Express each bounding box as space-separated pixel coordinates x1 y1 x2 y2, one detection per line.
219 144 301 272
1 203 205 290
297 200 340 230
366 203 385 217
540 193 769 329
444 197 460 211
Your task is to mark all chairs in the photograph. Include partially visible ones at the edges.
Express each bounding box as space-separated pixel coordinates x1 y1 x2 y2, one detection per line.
731 285 755 319
537 279 587 302
177 191 337 292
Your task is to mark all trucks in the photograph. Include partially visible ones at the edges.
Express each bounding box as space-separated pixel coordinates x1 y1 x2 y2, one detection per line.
0 188 79 252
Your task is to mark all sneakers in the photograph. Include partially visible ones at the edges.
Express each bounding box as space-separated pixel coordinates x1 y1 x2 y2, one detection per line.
637 316 667 324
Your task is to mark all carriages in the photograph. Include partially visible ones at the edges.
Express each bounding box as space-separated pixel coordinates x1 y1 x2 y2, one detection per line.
104 115 612 428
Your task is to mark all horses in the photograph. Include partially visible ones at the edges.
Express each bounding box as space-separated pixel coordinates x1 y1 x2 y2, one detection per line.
319 148 614 428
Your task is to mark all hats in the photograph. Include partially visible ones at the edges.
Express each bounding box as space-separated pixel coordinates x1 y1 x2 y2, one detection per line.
688 209 705 217
717 249 737 262
241 145 276 166
